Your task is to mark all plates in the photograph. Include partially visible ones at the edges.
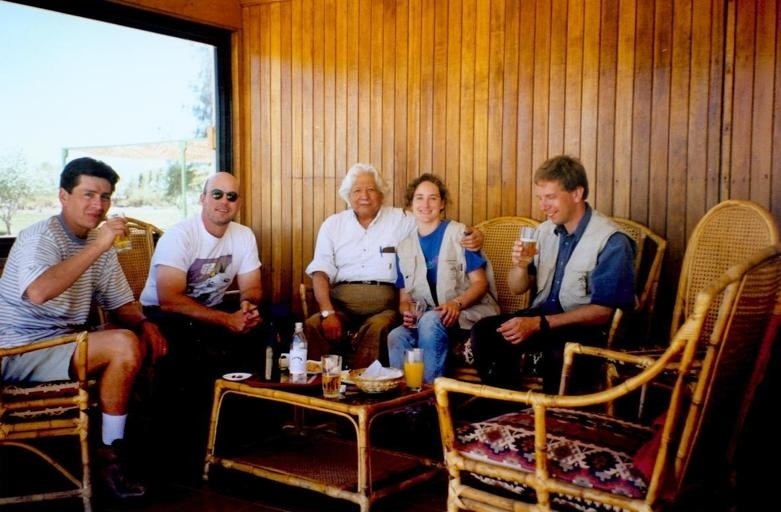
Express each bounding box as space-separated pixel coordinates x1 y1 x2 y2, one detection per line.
222 371 253 381
305 359 325 374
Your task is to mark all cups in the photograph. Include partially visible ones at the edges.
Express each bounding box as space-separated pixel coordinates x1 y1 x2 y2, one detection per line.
318 353 342 398
105 209 133 253
514 225 542 261
404 346 425 391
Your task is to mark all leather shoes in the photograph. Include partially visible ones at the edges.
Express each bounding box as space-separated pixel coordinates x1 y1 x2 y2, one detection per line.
92 438 148 500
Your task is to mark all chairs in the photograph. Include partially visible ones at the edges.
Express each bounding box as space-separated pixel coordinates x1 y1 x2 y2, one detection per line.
449 215 668 391
0 330 91 511
607 198 779 420
433 246 781 512
97 216 164 329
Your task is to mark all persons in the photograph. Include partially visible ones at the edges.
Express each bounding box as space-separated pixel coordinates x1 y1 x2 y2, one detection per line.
138 171 278 381
300 162 485 382
0 156 174 499
385 173 499 384
470 154 634 422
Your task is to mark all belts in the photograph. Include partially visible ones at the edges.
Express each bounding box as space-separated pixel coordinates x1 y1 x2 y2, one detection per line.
336 281 395 286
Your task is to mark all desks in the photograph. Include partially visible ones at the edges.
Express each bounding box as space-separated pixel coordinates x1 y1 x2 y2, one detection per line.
203 362 441 511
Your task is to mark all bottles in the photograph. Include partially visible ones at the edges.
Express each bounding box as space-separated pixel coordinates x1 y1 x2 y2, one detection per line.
263 328 280 384
289 322 309 376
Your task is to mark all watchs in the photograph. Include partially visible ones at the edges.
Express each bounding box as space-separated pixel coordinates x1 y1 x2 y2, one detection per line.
320 309 336 318
539 314 550 332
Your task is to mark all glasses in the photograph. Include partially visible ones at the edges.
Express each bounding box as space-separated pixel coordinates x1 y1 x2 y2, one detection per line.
212 189 238 202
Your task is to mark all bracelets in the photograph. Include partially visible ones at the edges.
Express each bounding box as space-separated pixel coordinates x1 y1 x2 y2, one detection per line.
453 297 463 308
135 319 151 329
